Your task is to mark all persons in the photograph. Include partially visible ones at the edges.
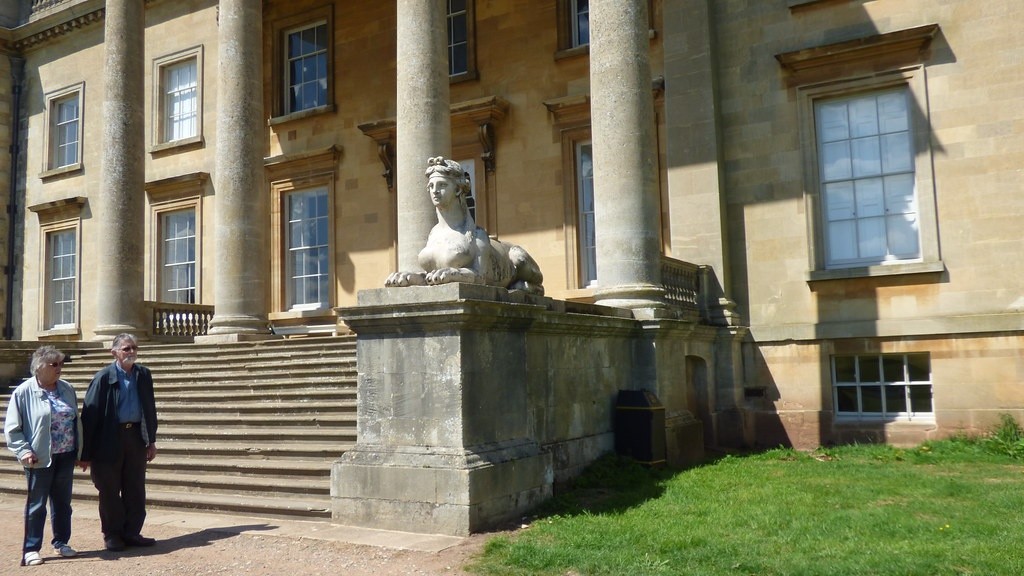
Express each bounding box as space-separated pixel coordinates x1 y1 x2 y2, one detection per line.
384 157 544 296
4 345 82 566
75 333 159 554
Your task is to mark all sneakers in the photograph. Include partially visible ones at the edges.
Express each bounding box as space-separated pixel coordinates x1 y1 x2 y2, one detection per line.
54 545 77 557
24 551 44 565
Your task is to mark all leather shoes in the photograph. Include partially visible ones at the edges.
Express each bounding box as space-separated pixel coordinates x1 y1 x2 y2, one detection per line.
104 535 126 550
124 534 156 545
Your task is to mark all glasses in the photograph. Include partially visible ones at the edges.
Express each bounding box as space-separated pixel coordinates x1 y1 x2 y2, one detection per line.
49 361 64 367
113 345 138 352
